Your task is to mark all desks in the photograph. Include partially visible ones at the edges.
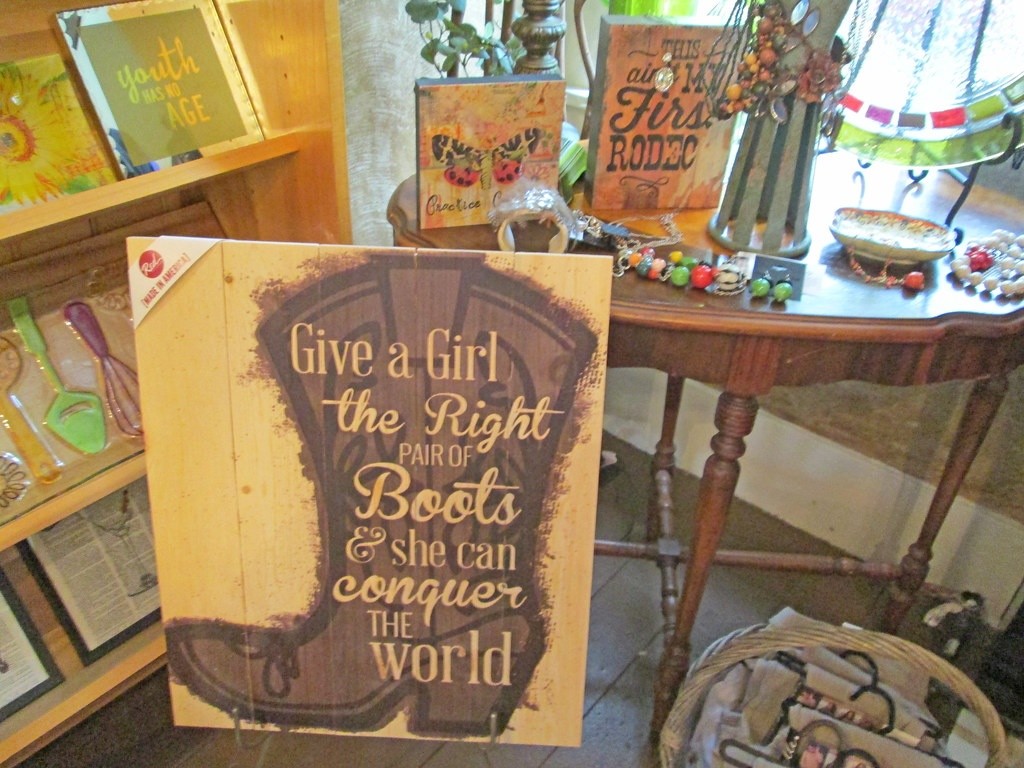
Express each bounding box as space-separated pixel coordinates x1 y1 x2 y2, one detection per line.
382 174 1024 768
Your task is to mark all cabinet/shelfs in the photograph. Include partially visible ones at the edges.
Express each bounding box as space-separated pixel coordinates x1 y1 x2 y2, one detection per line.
0 0 353 768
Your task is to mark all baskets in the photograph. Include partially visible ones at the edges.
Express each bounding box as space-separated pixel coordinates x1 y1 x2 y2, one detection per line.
659 620 1007 768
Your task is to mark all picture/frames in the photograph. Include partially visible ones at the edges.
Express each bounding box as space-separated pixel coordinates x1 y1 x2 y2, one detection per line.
50 0 266 176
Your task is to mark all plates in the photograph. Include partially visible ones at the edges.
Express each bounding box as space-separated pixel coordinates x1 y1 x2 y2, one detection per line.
830 207 958 265
